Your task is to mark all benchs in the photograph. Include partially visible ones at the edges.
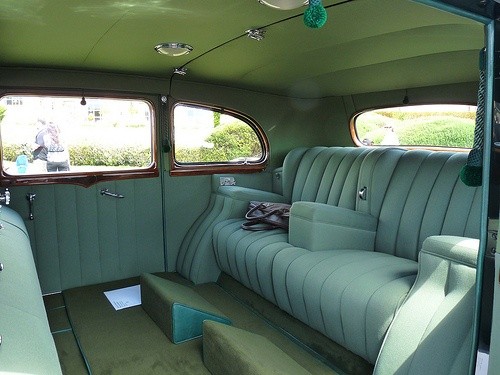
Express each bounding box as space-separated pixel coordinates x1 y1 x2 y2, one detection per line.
175 146 483 375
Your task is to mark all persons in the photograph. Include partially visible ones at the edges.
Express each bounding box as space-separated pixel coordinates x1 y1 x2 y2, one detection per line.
31 116 47 173
43 120 71 172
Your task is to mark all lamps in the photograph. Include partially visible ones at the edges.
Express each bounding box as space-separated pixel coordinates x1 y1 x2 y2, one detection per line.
154 42 193 56
258 0 309 10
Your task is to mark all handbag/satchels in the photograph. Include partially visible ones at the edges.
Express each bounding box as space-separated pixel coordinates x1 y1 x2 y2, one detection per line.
242 201 293 231
